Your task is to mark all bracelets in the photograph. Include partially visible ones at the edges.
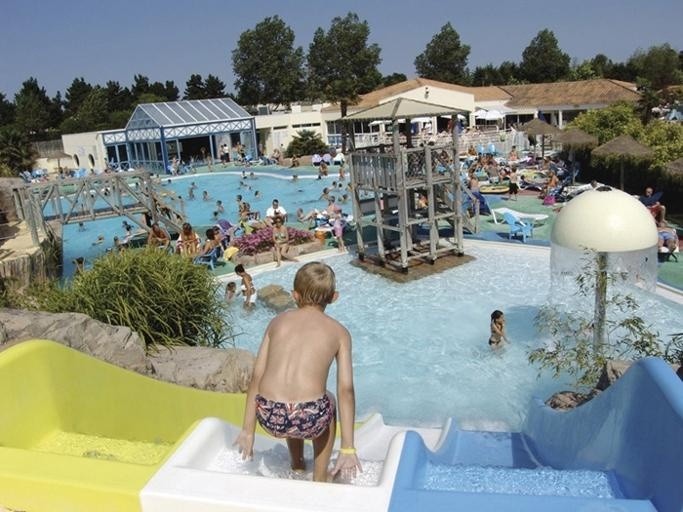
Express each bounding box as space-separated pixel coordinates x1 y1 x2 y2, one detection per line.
338 448 356 455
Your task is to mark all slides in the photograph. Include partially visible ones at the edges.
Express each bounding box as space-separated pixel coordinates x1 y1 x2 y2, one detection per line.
143 410 454 512
383 357 683 512
1 339 376 512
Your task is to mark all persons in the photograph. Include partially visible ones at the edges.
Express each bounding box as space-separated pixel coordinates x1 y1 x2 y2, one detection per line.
488 309 504 350
231 261 363 485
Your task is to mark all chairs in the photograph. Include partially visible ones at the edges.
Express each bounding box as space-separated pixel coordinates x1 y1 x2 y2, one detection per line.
489 206 549 245
193 248 219 272
